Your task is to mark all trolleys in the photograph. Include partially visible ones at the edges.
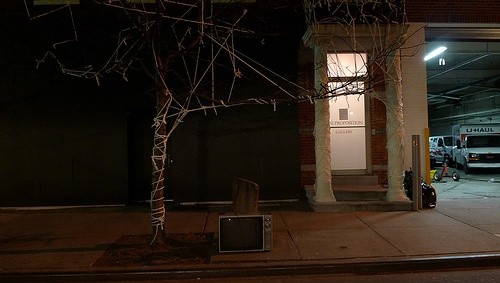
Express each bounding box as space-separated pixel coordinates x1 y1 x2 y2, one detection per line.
433 142 460 182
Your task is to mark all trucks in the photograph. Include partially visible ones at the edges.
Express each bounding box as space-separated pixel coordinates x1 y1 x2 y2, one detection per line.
453 121 498 174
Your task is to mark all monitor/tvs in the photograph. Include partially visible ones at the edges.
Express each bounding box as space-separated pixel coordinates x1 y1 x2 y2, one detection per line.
218 212 274 253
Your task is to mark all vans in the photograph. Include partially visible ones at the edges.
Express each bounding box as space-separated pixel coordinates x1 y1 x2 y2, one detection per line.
430 136 455 157
